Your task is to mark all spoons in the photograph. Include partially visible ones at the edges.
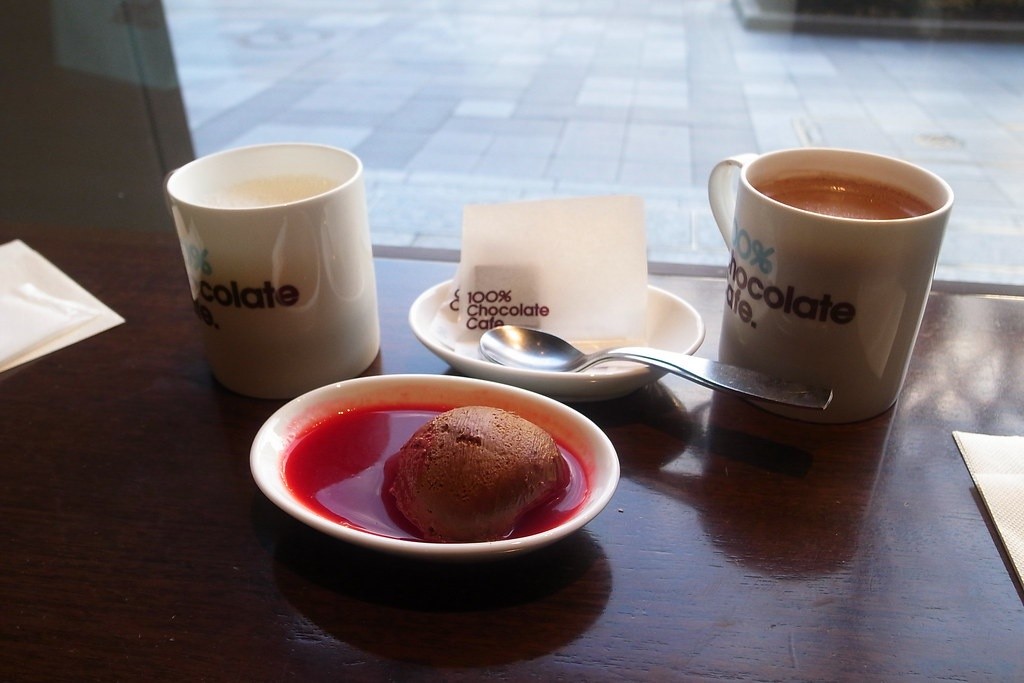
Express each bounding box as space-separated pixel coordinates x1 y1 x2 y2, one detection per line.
479 325 833 410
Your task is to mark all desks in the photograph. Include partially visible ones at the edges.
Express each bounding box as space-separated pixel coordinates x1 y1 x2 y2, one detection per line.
0 233 1023 682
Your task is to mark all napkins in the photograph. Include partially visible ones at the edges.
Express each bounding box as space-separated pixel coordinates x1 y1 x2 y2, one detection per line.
952 431 1023 588
1 239 127 374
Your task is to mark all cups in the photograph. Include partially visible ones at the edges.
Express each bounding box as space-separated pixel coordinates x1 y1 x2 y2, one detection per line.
707 148 954 425
163 143 379 401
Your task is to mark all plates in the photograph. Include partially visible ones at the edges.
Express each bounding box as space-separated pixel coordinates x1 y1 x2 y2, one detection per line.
408 277 706 403
249 374 620 554
